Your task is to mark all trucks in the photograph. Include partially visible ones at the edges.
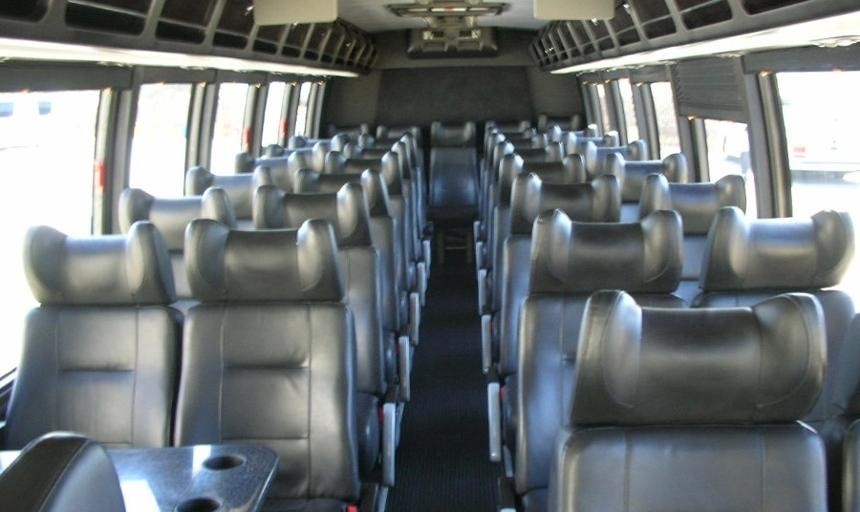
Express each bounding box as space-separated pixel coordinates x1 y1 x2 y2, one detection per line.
721 76 860 181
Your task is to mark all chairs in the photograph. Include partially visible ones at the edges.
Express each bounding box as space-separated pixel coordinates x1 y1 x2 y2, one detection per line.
427 117 476 269
